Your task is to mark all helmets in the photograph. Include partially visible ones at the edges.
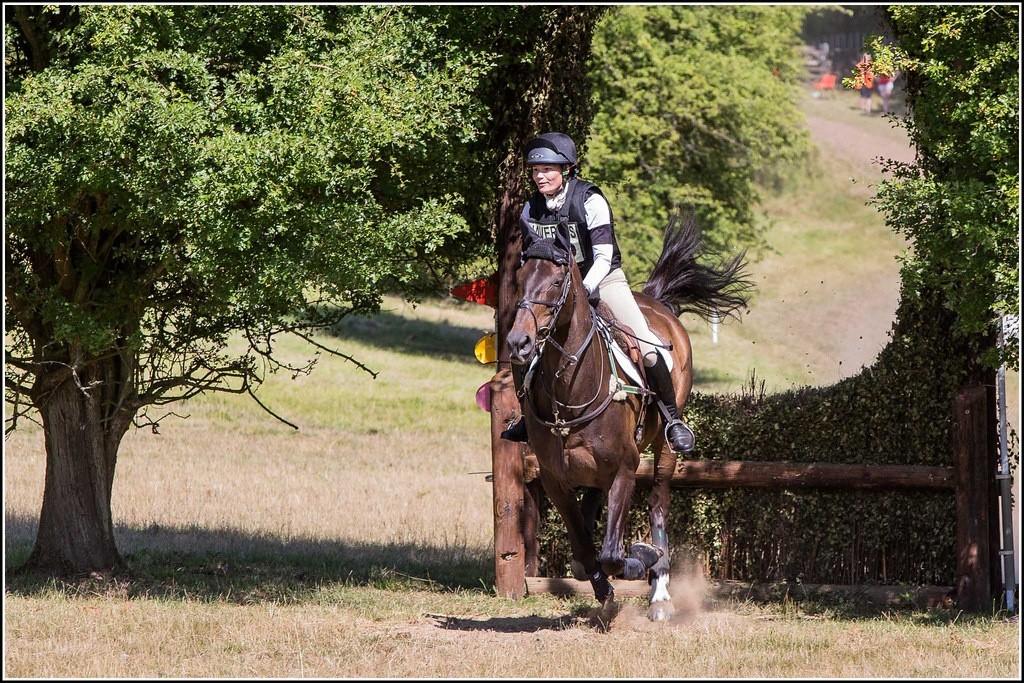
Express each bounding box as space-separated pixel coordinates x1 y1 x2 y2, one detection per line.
524 132 578 165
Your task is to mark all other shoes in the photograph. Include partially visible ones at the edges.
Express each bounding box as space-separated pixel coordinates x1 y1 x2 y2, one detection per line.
662 417 694 453
499 416 528 442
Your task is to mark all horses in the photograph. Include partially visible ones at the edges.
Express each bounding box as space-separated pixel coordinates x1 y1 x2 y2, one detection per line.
505 214 753 625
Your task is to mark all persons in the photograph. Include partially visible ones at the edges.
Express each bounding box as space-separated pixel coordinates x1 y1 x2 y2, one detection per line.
853 49 901 115
500 131 696 453
